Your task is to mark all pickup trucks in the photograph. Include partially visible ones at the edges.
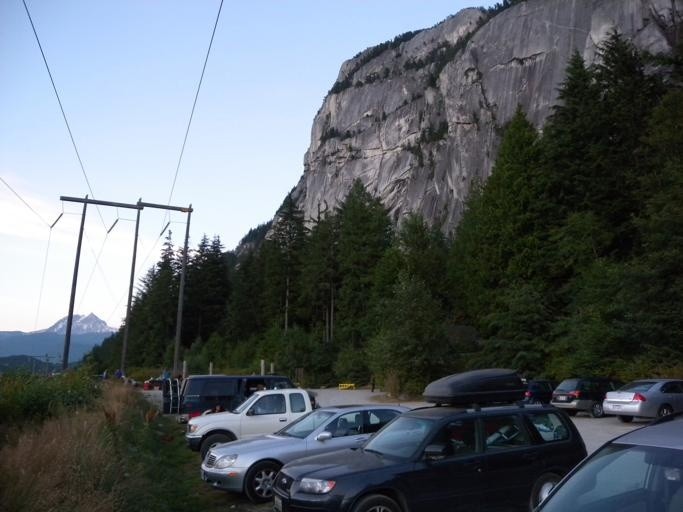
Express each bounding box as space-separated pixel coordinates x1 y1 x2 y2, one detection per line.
186 387 315 461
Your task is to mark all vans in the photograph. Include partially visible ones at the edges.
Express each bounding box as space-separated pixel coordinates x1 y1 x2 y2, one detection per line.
177 376 317 422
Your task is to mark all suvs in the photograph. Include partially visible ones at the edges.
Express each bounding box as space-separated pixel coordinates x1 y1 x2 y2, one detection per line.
274 367 589 512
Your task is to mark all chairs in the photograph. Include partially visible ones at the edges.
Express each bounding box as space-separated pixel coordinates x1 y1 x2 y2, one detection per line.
325 414 364 436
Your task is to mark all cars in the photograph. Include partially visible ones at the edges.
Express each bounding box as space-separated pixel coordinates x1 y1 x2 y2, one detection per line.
533 411 683 511
523 378 681 422
201 405 412 503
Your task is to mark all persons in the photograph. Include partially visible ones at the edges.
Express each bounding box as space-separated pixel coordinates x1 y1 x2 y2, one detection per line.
255 380 264 393
212 403 222 412
